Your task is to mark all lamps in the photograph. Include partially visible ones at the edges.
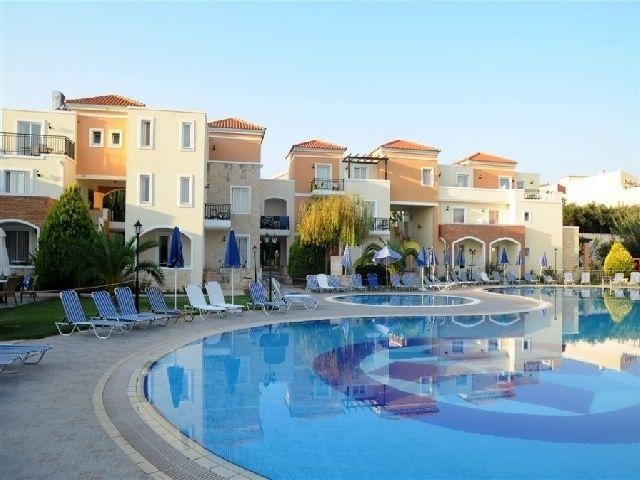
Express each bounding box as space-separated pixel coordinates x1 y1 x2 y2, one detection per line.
369 219 390 232
207 206 232 220
314 178 344 192
181 272 381 323
1 261 180 378
260 214 289 229
22 141 54 156
387 261 640 295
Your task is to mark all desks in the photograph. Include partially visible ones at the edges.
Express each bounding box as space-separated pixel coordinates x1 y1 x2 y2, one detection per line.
253 244 258 284
134 220 143 314
553 290 558 320
263 325 277 390
553 248 557 274
263 233 278 301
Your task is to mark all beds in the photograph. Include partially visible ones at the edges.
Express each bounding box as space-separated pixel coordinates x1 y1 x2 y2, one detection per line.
541 252 547 271
516 252 524 283
168 226 184 310
500 248 510 279
341 243 352 275
444 248 451 283
342 320 350 342
168 351 185 410
455 249 464 280
426 247 439 282
373 246 402 287
224 229 241 305
419 316 435 335
224 332 240 396
0 228 10 280
540 292 546 317
416 247 426 290
